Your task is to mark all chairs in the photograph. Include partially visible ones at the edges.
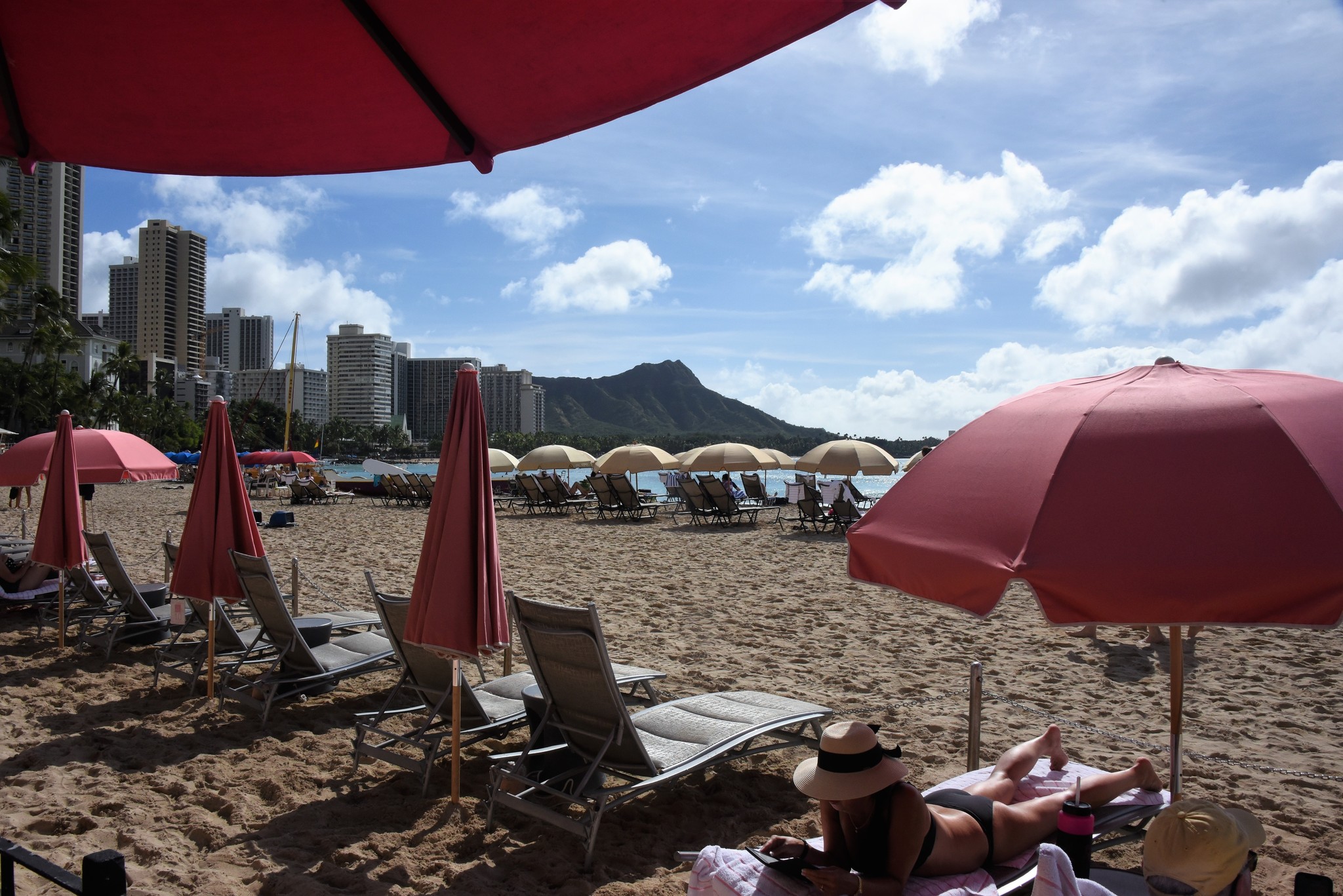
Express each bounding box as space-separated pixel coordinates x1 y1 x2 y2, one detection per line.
0 531 1169 896
219 464 882 527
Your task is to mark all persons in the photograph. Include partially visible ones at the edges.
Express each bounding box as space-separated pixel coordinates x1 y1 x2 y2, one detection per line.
14 484 32 510
752 473 778 497
590 471 596 477
9 485 22 508
918 446 933 461
323 452 428 477
321 487 354 496
729 477 741 491
522 473 527 476
554 474 591 496
0 550 106 594
720 473 749 499
758 720 1166 896
1140 795 1268 896
541 470 548 477
319 476 331 487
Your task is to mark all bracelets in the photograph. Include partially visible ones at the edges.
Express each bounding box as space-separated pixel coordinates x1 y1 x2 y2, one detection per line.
854 875 864 896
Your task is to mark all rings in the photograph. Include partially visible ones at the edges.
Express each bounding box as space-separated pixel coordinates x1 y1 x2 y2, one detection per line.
819 885 824 892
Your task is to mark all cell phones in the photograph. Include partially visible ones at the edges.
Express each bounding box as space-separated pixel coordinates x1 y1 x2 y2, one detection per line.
743 845 784 865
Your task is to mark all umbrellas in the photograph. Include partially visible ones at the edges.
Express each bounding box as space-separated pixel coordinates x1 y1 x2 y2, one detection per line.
678 442 778 497
566 450 596 491
794 437 900 488
593 438 711 493
0 427 181 571
516 442 592 496
162 448 319 490
902 446 937 471
758 446 797 491
488 444 520 473
846 358 1341 813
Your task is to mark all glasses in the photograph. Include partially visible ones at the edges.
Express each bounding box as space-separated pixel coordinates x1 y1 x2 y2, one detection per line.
1231 849 1258 896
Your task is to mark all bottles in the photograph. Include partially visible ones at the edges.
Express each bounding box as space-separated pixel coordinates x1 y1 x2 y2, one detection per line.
1055 800 1095 884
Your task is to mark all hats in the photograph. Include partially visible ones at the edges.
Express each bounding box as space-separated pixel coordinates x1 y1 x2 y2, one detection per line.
1142 799 1267 895
794 722 908 805
